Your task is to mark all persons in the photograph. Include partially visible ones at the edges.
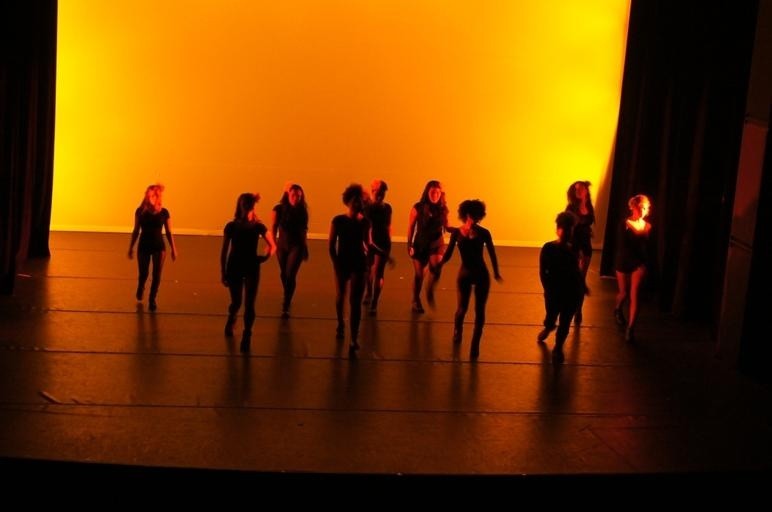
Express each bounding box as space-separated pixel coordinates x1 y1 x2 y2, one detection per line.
328 184 398 351
267 183 309 321
612 193 654 343
428 199 506 362
127 184 177 310
362 181 392 316
221 193 277 357
565 181 597 324
536 211 591 363
406 180 458 314
